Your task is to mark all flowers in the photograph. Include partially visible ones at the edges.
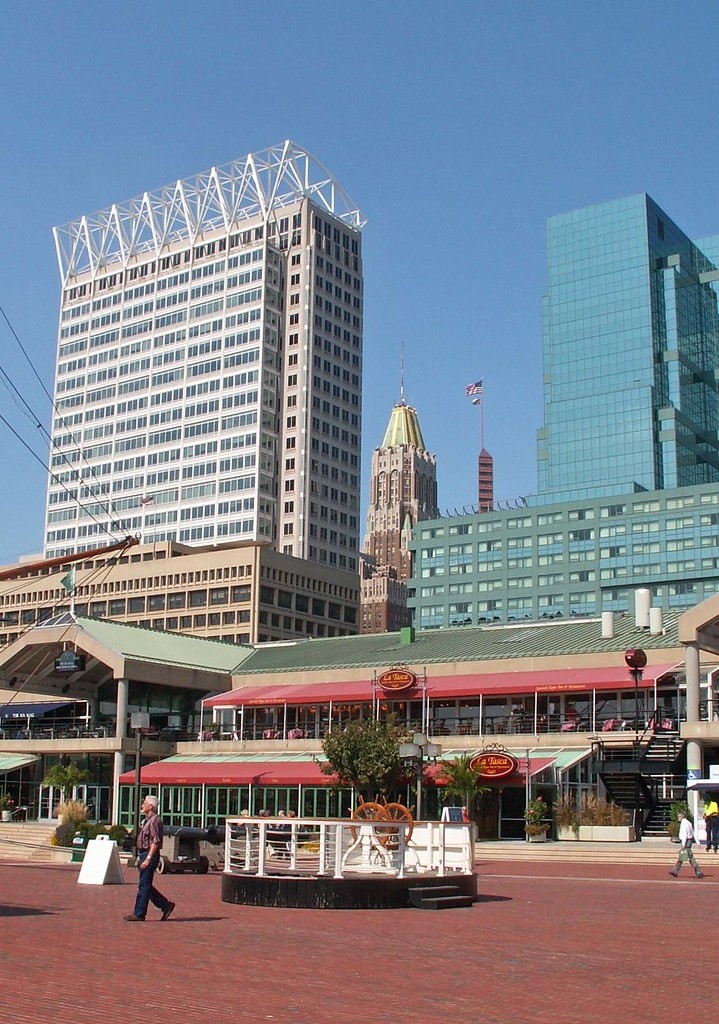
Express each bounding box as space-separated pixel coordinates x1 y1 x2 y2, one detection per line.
524 796 548 824
0 792 14 812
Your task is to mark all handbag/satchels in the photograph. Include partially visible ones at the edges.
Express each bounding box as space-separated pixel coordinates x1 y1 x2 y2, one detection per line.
679 849 688 861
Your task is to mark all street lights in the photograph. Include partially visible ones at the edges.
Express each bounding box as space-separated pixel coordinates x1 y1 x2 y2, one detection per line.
400 737 442 821
126 711 152 868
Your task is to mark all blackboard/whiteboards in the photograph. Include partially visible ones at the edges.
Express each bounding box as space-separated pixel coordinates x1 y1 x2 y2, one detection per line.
446 807 463 822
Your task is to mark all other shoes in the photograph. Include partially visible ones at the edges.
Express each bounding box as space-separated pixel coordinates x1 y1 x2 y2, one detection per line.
668 871 677 877
697 873 704 878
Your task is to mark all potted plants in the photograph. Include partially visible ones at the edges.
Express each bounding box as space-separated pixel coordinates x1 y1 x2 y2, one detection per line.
524 824 550 843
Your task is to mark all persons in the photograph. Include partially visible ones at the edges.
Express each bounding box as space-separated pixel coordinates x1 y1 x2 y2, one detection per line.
22 725 27 735
702 794 719 850
568 704 576 721
513 703 525 718
648 716 668 728
239 809 295 859
123 796 175 921
669 812 703 878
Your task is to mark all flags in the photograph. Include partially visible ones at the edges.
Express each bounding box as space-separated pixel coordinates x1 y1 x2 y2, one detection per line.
142 496 155 505
473 398 481 404
61 569 74 592
465 380 482 395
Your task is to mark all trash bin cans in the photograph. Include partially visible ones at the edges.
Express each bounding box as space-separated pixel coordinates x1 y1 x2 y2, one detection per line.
71 832 86 861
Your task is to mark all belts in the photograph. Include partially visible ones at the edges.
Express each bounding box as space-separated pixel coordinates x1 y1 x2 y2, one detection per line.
139 849 149 853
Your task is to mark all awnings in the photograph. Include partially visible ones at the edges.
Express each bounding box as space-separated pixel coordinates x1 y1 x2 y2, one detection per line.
0 702 73 719
199 661 684 743
119 758 557 841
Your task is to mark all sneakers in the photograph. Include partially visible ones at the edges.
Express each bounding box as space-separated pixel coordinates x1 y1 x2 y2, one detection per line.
161 902 175 920
123 913 145 921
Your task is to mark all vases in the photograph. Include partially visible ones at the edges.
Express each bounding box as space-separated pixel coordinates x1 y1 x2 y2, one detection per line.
1 811 13 822
